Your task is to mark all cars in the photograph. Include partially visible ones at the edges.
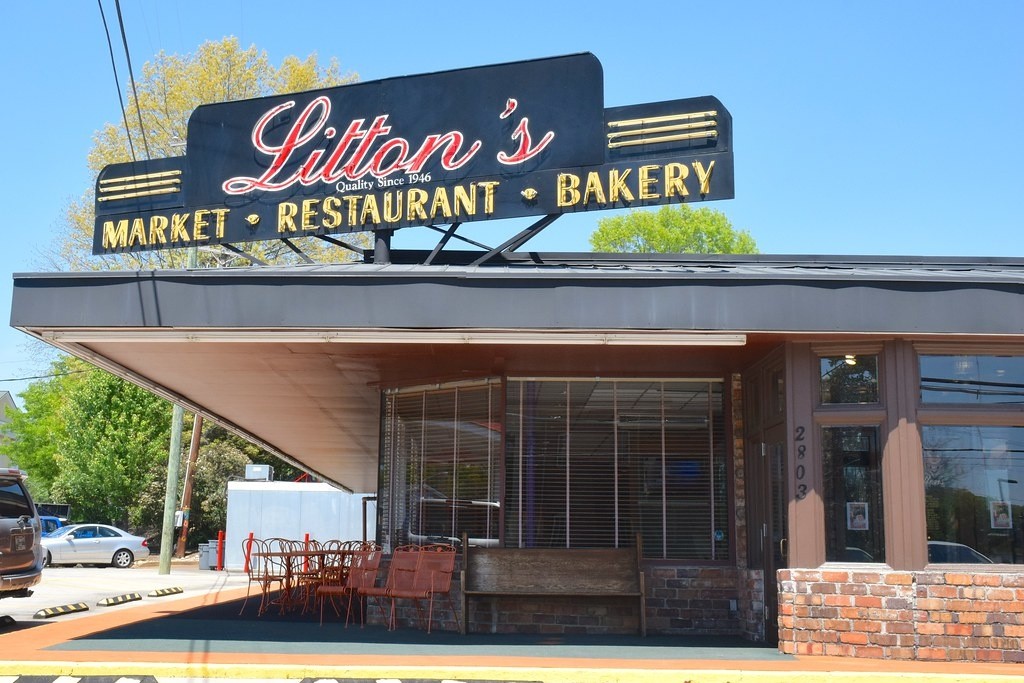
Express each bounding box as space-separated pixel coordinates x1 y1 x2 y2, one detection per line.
927 540 995 564
40 523 151 568
845 547 874 562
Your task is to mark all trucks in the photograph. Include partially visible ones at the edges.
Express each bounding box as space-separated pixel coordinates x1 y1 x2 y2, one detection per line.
39 515 102 568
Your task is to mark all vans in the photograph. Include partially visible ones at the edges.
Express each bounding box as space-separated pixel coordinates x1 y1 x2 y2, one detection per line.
410 497 500 547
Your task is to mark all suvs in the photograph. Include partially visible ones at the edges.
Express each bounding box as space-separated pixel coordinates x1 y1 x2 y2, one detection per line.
0 467 46 591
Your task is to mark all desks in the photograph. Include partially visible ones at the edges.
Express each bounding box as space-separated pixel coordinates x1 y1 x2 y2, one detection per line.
253 550 359 615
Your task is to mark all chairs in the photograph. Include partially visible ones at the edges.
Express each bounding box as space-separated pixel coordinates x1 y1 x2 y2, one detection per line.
358 543 463 635
242 538 383 629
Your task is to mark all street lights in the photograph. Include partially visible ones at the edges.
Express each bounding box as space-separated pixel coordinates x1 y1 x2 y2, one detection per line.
997 477 1018 563
